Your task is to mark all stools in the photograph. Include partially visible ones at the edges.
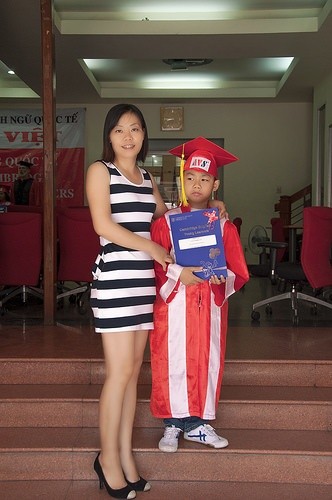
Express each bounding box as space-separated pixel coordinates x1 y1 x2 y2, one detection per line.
258 241 289 286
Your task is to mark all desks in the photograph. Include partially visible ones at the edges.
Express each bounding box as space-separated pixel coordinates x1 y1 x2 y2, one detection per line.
281 225 304 263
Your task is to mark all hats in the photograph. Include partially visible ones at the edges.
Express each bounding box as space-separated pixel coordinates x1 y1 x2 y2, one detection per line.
168 136 239 207
17 161 34 168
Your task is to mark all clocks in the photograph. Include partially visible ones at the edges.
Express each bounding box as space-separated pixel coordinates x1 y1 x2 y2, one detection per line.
160 106 184 131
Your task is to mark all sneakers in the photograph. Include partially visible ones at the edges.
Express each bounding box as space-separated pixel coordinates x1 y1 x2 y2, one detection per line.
184 423 229 449
158 424 184 453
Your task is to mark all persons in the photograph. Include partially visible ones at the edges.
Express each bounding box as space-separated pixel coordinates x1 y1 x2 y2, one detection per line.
86 102 229 500
10 160 42 206
149 134 251 454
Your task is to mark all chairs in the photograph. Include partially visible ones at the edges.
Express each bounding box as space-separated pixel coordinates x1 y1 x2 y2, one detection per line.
56 206 102 310
251 207 332 326
0 212 43 307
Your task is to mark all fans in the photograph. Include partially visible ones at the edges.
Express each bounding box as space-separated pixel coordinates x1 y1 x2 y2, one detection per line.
248 225 270 264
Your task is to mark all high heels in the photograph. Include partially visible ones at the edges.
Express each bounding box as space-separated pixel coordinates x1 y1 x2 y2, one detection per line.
122 468 152 492
93 450 136 500
